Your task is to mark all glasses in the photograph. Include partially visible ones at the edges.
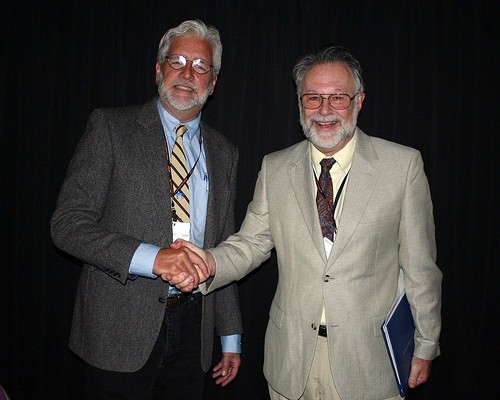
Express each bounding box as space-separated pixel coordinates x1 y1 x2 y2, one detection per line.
164 54 215 74
300 92 359 110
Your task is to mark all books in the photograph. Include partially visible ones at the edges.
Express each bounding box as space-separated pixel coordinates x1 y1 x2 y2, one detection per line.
381 289 422 398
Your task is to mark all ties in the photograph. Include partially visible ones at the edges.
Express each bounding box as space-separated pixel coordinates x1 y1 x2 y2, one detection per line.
170 125 191 222
316 157 336 242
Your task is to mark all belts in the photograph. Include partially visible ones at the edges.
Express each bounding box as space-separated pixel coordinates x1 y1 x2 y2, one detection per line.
318 325 327 337
166 294 201 308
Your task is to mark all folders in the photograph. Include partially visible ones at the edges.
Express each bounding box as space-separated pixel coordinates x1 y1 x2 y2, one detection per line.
381 288 415 398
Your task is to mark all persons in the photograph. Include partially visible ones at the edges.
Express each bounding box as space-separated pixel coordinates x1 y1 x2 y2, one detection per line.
161 43 444 400
49 17 241 400
